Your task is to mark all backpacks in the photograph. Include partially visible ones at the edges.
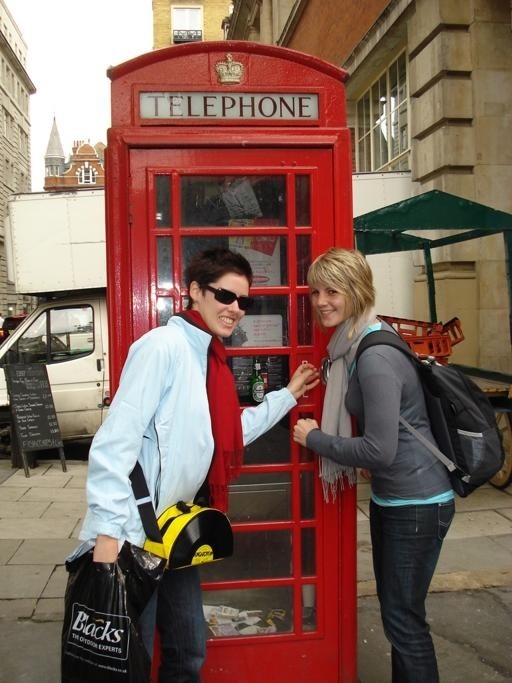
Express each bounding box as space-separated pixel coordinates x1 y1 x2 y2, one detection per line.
353 313 506 497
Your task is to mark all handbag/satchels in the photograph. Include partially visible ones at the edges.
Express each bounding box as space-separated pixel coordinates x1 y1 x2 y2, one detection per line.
144 496 235 575
59 534 159 683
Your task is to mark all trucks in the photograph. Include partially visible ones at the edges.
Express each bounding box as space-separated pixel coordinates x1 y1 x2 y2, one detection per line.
1 167 418 466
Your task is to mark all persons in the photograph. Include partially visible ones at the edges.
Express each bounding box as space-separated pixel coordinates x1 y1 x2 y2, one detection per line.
289 246 463 681
71 242 321 683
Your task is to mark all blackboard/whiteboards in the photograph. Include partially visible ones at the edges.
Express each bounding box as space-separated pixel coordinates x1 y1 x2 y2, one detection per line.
3 361 64 452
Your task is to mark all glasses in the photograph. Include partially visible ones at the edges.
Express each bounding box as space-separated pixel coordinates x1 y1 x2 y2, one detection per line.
320 356 332 385
200 285 255 310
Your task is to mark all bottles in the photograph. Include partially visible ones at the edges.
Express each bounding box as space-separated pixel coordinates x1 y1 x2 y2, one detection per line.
249 359 266 406
